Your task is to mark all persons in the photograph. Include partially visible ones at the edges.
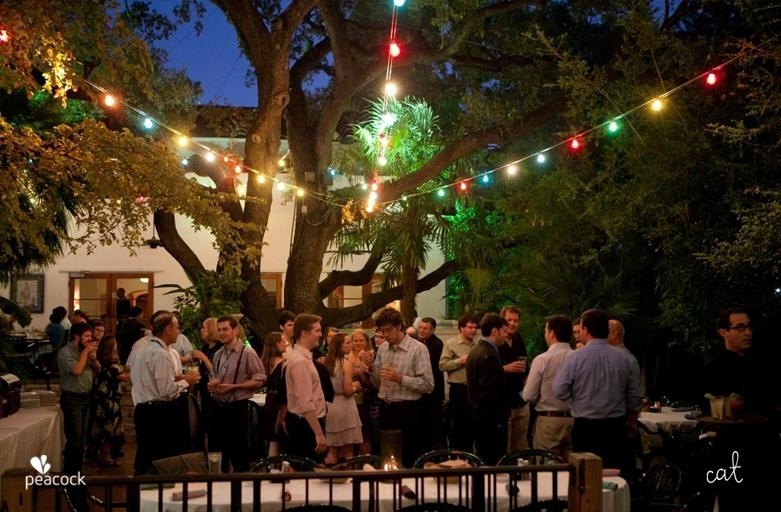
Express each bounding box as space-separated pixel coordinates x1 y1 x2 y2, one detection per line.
38 280 654 478
690 309 776 512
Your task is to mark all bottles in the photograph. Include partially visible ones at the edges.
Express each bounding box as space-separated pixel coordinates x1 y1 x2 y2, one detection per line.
518 458 531 481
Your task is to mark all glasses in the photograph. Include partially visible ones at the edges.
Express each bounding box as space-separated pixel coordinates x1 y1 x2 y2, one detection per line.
726 322 753 331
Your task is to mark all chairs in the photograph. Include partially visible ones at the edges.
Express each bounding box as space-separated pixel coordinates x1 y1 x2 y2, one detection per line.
2 331 36 376
60 448 575 510
188 393 209 441
245 399 264 445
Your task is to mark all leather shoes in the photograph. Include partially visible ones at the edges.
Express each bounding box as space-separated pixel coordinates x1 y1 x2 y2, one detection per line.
102 460 122 466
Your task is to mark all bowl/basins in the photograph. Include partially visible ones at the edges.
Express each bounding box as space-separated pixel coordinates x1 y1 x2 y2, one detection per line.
314 467 352 484
425 463 463 483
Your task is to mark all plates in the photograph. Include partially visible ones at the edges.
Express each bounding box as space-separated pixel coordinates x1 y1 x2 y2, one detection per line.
21 390 57 408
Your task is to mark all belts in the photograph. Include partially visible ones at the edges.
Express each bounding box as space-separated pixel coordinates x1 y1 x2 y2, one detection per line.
540 410 572 418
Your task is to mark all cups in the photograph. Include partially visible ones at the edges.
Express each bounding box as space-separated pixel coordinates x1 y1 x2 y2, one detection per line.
518 356 528 372
187 362 200 384
208 374 221 395
208 453 223 474
